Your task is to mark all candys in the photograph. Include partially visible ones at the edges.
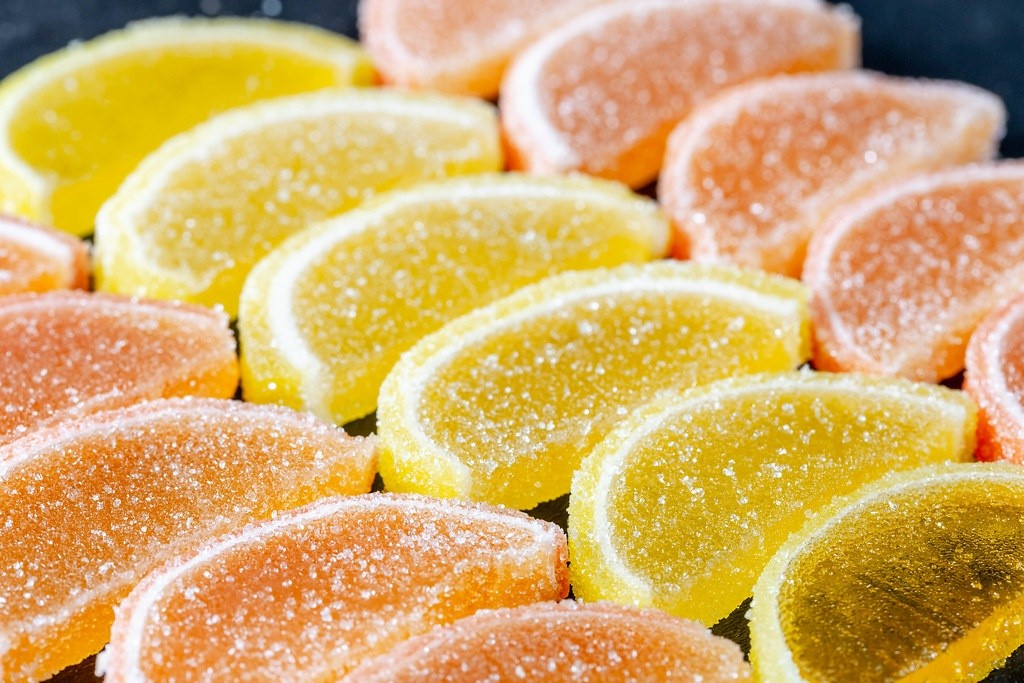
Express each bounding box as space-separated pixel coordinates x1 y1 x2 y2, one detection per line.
0 1 1024 683
